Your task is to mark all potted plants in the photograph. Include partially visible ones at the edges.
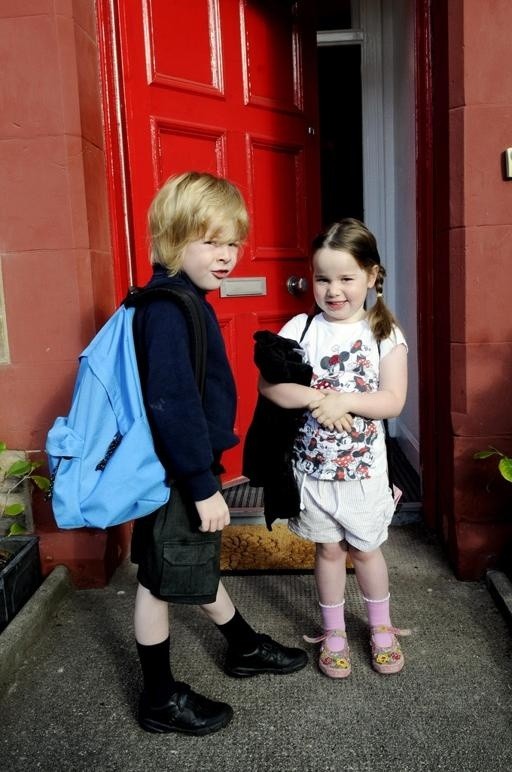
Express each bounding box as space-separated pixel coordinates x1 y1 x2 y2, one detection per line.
0 440 52 634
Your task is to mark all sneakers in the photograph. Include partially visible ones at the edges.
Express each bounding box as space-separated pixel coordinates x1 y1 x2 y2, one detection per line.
224 631 311 679
136 680 235 737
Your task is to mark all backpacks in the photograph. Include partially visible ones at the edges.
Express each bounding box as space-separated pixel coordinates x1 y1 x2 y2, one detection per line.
43 282 210 535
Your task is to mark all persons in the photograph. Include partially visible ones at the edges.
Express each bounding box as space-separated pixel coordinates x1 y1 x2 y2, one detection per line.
113 169 309 738
255 212 411 683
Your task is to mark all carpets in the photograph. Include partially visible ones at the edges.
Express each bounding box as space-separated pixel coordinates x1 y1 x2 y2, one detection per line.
217 522 356 576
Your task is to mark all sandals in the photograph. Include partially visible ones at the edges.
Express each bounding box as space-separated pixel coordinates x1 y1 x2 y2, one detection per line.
318 627 353 679
367 621 405 676
